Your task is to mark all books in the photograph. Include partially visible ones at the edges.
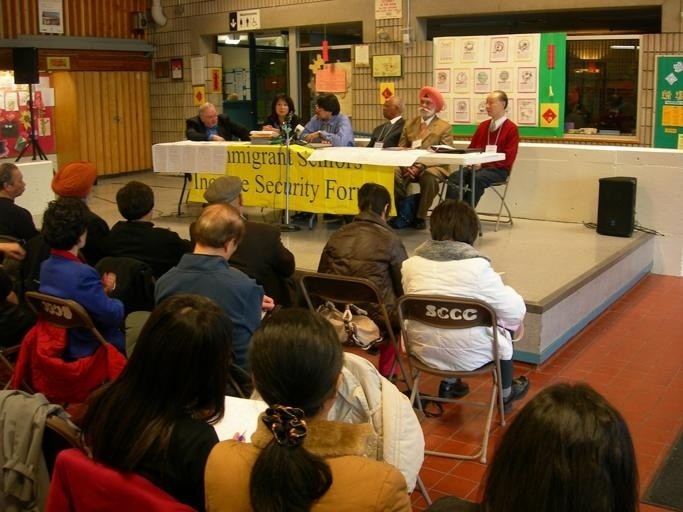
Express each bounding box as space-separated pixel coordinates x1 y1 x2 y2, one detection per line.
249 130 286 145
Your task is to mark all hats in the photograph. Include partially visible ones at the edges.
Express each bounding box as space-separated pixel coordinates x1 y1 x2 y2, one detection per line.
419 84 446 114
203 175 243 206
50 158 99 198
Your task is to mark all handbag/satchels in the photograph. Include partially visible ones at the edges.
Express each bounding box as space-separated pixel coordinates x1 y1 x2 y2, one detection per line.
315 300 383 352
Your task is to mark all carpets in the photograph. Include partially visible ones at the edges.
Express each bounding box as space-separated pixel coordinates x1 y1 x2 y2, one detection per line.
639 404 683 512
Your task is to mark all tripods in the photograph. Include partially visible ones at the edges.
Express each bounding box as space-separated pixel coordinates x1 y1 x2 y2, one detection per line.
14 85 49 162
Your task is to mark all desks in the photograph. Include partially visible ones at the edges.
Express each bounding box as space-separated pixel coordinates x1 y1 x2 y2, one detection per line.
151 140 506 238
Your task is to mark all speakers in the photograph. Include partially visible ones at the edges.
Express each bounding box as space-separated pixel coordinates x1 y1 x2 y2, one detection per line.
598 177 638 238
12 50 40 84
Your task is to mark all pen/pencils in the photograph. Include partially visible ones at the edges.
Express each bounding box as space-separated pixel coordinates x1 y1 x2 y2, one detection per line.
238 429 247 443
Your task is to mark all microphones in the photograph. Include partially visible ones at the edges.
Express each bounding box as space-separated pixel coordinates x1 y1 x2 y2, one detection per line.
284 111 294 124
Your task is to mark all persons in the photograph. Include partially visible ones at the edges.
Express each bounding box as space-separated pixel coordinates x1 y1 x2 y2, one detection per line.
1 161 527 512
256 92 306 143
185 102 252 142
291 93 356 221
446 90 519 209
394 85 453 229
425 377 642 512
365 94 406 148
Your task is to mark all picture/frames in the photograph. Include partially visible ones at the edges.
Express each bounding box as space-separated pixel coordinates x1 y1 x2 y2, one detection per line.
171 58 183 80
432 36 537 125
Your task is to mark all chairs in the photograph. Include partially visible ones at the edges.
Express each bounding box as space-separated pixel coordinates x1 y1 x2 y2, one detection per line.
411 179 446 218
477 174 513 232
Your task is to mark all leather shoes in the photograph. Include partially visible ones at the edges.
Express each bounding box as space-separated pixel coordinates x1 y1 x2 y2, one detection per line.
495 374 530 414
438 380 469 399
291 210 319 223
412 217 427 230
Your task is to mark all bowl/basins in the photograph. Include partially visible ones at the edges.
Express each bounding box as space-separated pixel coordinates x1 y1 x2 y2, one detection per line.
452 140 471 148
354 138 371 146
